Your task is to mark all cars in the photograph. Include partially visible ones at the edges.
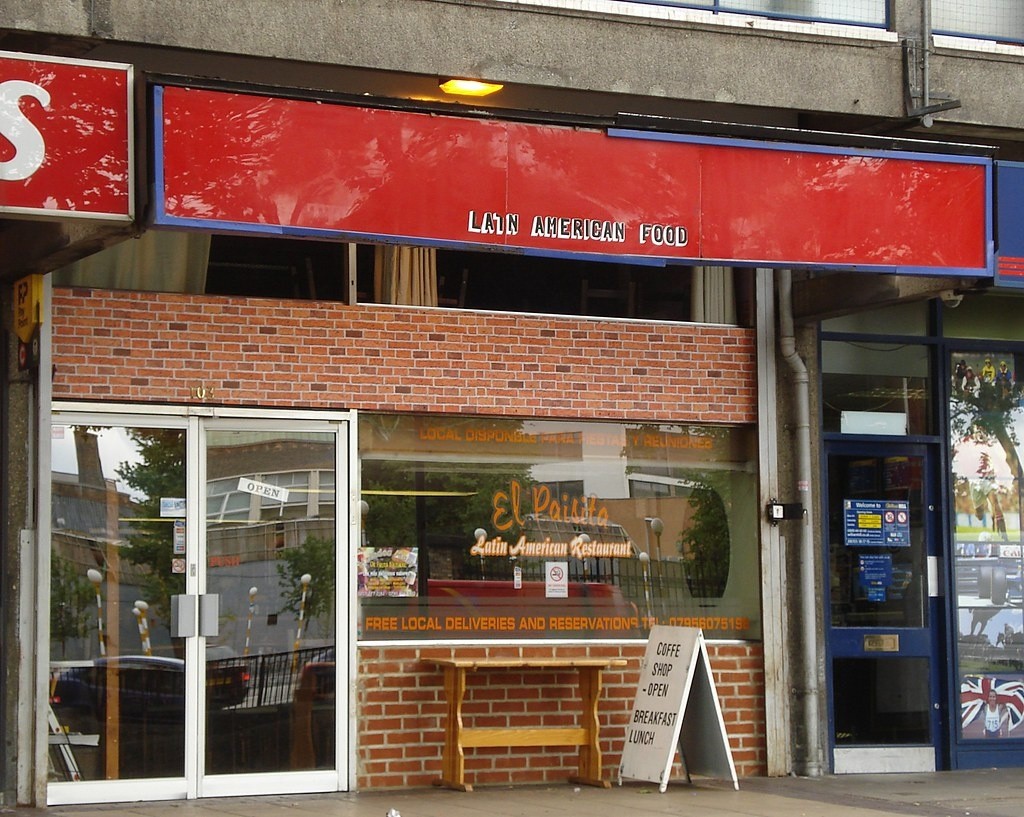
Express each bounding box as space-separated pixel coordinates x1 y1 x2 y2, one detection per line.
46 653 248 725
293 647 336 702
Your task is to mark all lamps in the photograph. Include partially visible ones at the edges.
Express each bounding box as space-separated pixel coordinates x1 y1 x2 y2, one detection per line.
437 79 504 97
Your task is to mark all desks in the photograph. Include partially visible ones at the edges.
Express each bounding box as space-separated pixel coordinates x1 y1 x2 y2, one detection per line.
419 657 624 793
